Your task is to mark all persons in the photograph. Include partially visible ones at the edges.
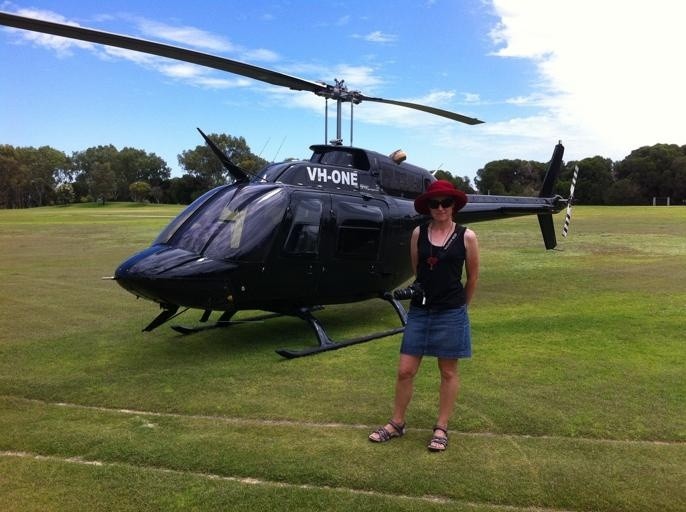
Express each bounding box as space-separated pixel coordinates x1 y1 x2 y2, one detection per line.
368 175 480 451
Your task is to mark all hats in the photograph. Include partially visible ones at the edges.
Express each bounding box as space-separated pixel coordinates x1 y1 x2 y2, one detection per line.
414 180 468 215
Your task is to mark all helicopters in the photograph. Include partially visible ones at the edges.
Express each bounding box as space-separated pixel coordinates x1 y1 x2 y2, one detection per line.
0 12 580 360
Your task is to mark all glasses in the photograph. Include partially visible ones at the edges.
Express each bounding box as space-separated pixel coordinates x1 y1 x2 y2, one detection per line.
427 197 455 209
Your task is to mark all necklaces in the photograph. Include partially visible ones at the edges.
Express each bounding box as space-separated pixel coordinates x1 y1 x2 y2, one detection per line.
425 221 455 270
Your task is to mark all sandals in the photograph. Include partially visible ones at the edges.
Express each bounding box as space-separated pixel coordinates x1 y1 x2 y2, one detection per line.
427 424 448 451
368 417 407 443
